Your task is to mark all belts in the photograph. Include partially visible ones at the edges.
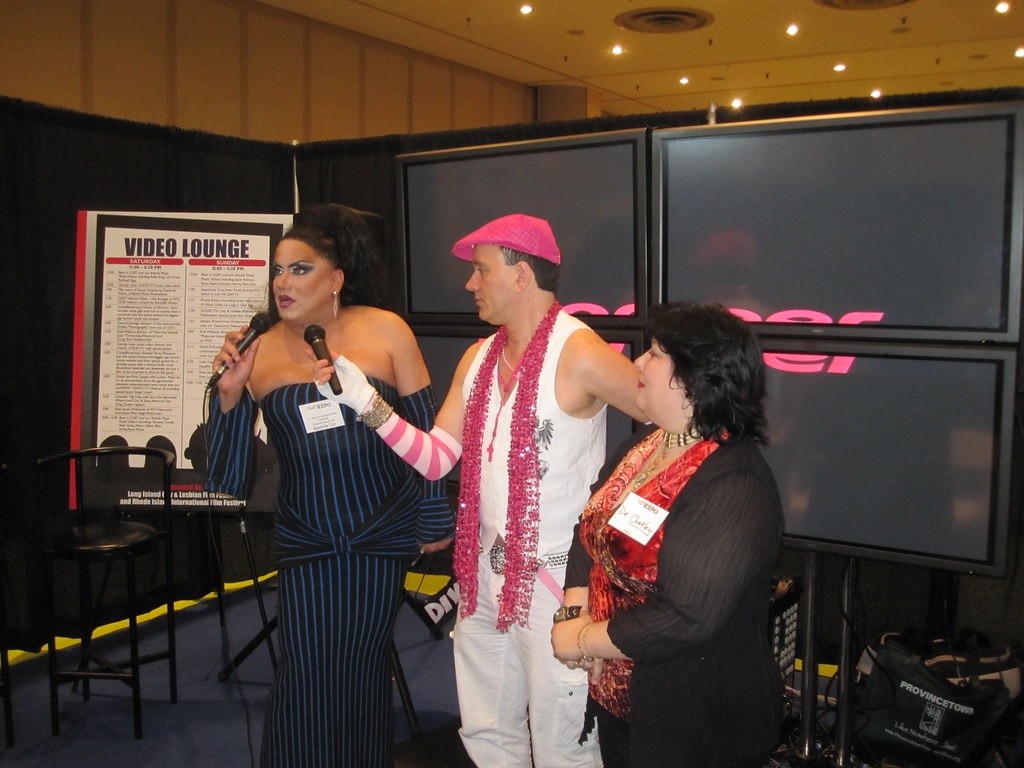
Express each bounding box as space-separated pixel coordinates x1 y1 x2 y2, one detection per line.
490 545 570 574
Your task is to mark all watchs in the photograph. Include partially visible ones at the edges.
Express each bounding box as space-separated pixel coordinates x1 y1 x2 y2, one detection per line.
553 606 581 624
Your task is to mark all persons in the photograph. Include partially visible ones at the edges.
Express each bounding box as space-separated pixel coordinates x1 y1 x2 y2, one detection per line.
204 205 456 768
551 304 782 768
307 215 649 768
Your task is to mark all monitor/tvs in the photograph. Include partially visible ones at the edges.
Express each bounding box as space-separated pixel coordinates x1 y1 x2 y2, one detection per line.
396 101 1024 576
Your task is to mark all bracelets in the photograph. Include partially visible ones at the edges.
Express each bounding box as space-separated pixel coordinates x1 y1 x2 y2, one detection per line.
360 396 394 430
578 624 594 661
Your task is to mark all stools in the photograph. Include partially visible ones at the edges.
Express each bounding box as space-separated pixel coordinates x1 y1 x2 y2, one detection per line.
37 446 175 739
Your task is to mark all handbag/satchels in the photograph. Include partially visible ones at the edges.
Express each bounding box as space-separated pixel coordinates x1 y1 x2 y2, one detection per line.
848 622 1019 766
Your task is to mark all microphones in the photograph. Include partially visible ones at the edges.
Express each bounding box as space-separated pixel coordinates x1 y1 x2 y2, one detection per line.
303 324 343 395
206 312 271 390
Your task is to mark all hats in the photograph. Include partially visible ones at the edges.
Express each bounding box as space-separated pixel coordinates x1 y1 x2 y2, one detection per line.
450 213 560 266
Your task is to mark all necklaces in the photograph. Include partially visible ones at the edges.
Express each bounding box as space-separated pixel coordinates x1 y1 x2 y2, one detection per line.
635 450 666 488
487 359 524 461
502 349 520 380
663 430 700 447
